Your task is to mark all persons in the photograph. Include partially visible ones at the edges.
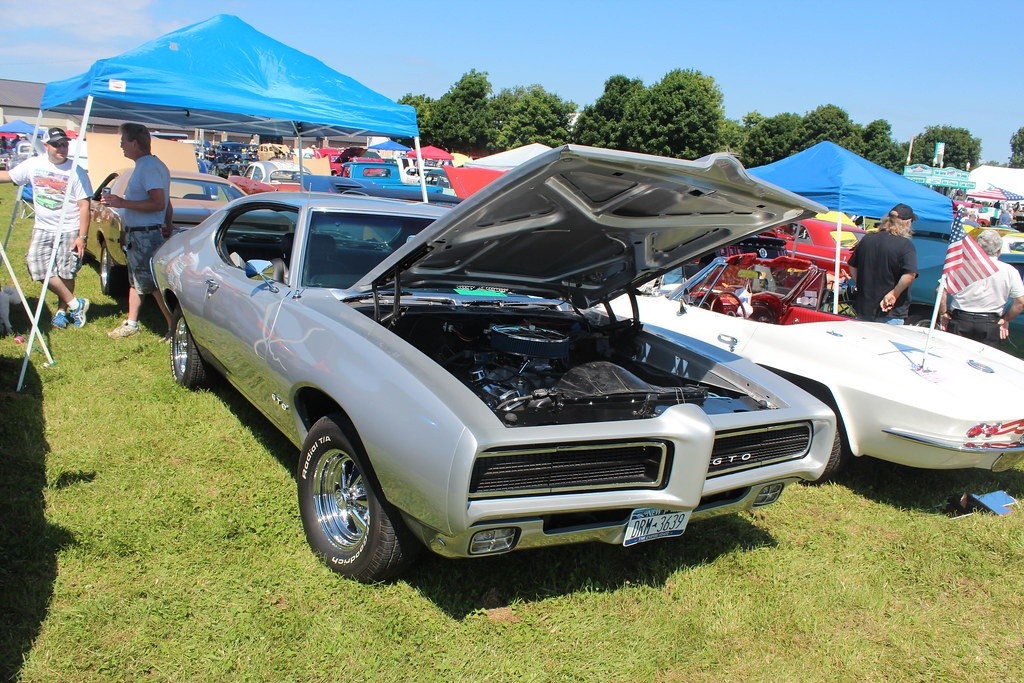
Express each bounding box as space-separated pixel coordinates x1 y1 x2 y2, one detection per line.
0 127 90 329
0 133 24 155
937 201 1024 351
100 123 173 345
848 203 919 328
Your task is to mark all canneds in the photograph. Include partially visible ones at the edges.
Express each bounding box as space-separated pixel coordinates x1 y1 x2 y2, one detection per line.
102 188 111 205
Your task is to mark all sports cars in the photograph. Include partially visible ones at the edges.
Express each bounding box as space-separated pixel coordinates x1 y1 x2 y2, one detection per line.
591 249 1023 482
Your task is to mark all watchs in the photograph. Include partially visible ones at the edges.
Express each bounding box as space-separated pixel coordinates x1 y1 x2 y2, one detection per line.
80 235 88 240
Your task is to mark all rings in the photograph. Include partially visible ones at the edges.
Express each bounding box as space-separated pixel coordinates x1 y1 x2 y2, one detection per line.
109 201 112 205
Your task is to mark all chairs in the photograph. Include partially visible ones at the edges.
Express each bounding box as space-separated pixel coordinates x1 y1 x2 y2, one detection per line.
281 232 338 275
182 193 211 200
15 183 35 219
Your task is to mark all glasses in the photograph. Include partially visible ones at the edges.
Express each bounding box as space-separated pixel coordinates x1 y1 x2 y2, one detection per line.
47 141 69 148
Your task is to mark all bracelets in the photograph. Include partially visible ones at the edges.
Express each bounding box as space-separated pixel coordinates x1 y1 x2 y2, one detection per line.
940 310 948 315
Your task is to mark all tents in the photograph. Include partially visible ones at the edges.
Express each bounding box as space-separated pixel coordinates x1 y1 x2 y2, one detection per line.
0 119 44 141
740 141 953 315
364 140 554 171
0 12 430 397
967 164 1024 208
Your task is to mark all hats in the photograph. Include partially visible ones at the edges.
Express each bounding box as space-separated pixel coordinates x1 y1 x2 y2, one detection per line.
43 128 71 143
889 203 918 221
957 190 963 195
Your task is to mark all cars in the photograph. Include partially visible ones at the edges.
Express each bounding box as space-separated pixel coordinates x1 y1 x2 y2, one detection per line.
0 140 38 187
775 218 868 284
997 235 1024 278
228 158 313 195
683 234 789 281
83 168 252 299
314 147 457 197
152 139 845 584
198 141 289 176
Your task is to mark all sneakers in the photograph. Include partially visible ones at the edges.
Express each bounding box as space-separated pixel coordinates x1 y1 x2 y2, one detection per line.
159 328 172 345
69 298 90 330
50 312 69 330
107 320 141 340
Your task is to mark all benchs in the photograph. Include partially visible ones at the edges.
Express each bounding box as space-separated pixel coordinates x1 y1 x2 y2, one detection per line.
229 242 388 274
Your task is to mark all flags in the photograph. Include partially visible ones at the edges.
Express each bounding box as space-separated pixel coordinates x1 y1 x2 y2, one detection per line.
942 207 1000 296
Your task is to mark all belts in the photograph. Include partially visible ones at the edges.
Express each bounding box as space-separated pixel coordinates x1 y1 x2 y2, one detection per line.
953 309 989 317
125 224 161 232
